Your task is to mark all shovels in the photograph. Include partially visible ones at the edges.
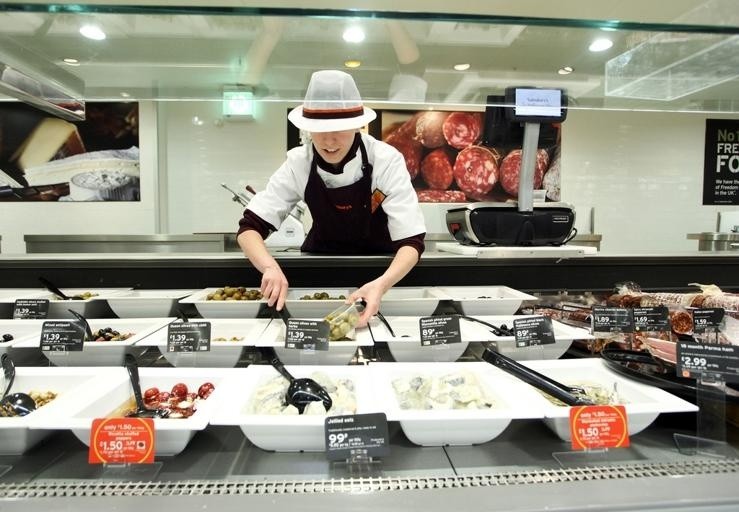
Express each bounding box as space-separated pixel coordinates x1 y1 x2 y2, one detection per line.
270 359 332 413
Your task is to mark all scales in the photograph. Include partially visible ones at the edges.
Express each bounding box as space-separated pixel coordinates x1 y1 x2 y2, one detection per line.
446 86 577 246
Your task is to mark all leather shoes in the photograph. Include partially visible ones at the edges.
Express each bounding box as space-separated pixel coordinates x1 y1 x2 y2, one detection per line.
287 70 377 132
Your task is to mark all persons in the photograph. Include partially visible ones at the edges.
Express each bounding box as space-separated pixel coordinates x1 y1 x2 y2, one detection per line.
236 69 427 331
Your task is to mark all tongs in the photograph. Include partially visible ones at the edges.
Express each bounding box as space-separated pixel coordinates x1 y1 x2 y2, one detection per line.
479 347 593 409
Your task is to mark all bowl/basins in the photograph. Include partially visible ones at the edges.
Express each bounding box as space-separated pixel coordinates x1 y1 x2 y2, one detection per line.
85 283 203 321
369 361 544 448
255 319 375 362
469 316 605 361
363 286 452 315
276 287 360 318
1 318 84 355
521 357 699 455
433 286 540 315
209 367 391 453
1 285 131 319
0 364 125 459
166 282 272 319
28 364 249 465
363 315 498 362
325 299 361 341
11 317 178 366
131 318 273 367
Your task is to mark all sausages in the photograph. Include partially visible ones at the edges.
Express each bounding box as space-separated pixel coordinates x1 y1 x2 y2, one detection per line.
537 291 739 351
384 110 562 203
